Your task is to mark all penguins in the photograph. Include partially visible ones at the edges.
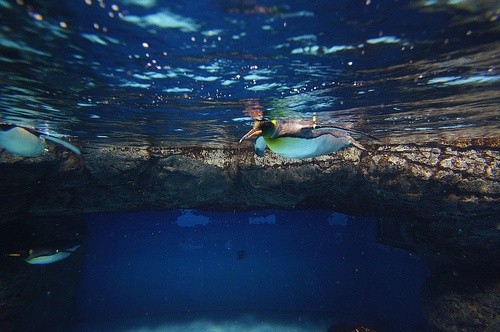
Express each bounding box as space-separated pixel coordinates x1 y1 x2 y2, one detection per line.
9 244 81 264
239 119 381 160
0 122 80 160
